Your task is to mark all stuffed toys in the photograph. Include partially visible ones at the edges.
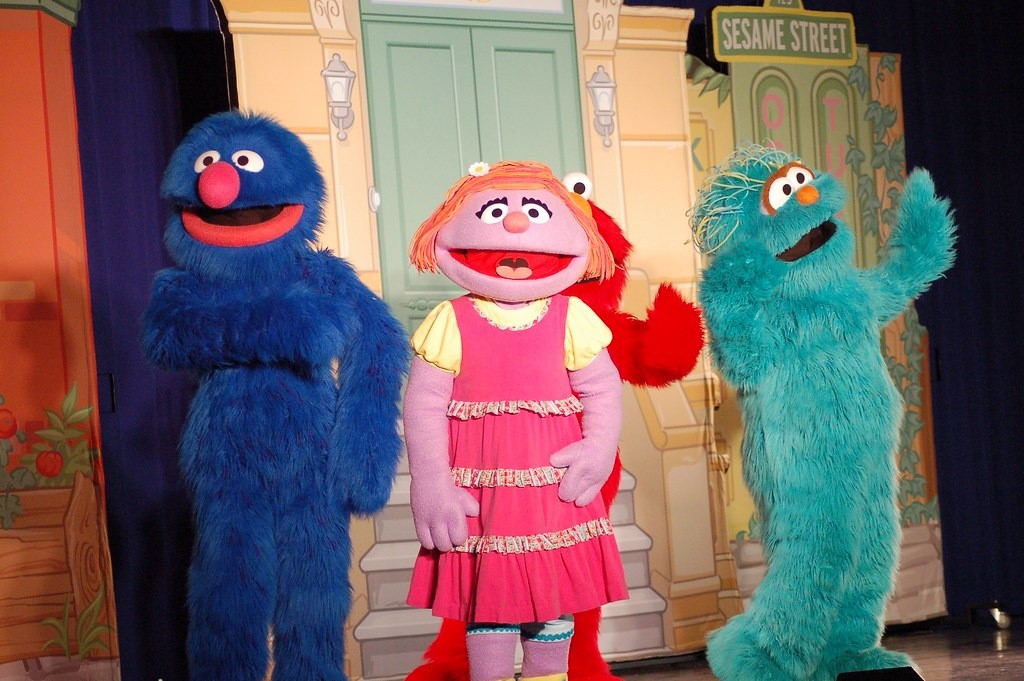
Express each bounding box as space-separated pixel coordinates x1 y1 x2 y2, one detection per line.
402 160 623 681
683 137 958 681
137 113 411 681
404 171 704 681
